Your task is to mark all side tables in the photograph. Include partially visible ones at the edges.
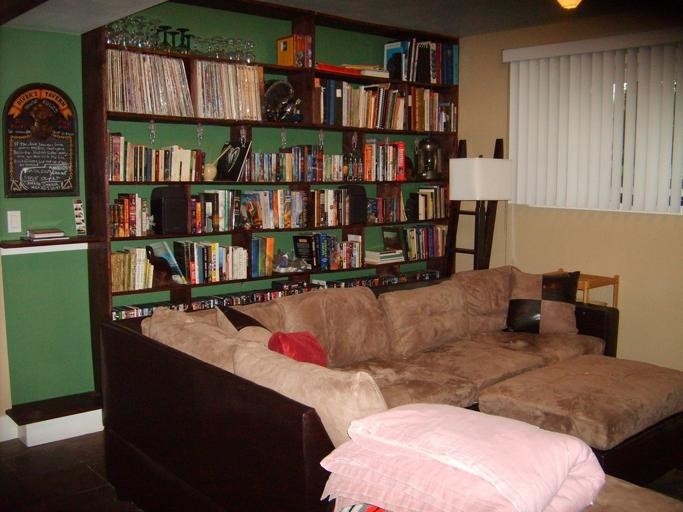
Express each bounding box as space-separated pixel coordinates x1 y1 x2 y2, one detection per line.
577 274 618 308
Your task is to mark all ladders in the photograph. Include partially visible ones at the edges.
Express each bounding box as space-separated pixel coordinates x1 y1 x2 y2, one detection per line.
448 138 504 274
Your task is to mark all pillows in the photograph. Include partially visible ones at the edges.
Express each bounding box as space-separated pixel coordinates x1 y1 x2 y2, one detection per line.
267 330 331 369
502 271 580 336
213 303 272 343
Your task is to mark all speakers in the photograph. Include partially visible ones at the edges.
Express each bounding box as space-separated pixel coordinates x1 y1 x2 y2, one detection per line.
338 184 368 225
150 187 188 234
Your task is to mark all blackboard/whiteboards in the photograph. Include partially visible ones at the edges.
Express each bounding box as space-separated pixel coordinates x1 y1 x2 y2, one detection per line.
4 132 79 198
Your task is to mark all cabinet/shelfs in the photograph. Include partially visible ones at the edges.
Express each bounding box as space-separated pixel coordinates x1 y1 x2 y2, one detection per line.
80 0 460 397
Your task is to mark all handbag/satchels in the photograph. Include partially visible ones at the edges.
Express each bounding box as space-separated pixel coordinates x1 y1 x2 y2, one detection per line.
146 245 172 288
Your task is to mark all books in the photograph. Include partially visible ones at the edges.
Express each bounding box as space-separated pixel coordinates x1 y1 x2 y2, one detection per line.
252 235 275 277
149 241 249 285
27 228 64 239
400 270 440 285
191 279 309 311
383 37 459 86
214 140 362 183
365 225 448 265
30 235 69 243
315 63 389 78
111 299 186 322
311 273 399 292
191 184 449 233
311 78 457 132
364 140 405 181
105 49 264 121
107 133 205 181
292 232 362 272
108 192 150 238
111 245 154 293
276 36 313 69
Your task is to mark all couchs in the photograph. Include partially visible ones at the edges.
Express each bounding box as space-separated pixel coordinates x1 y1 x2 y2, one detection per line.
99 271 618 511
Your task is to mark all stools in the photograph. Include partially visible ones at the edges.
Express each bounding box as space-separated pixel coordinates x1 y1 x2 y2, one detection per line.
480 355 683 486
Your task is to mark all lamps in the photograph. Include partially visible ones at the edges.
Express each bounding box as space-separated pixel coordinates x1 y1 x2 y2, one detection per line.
557 0 581 9
448 155 516 269
